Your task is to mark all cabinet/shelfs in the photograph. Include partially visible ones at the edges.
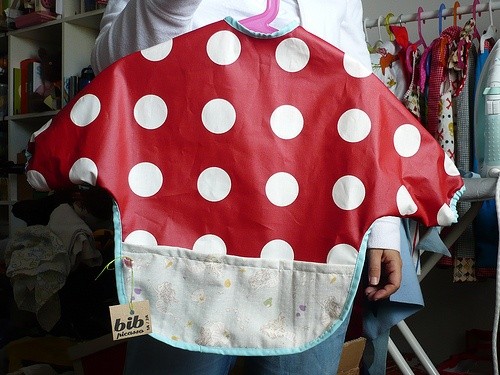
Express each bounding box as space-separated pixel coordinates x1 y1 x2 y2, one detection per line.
0 0 113 255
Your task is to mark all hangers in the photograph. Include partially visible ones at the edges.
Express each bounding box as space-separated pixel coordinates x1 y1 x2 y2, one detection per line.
361 1 497 76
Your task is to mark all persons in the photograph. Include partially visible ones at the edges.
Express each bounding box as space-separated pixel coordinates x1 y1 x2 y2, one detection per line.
85 0 404 374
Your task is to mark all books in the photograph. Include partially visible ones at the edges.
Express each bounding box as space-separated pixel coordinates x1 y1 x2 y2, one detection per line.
12 59 98 116
